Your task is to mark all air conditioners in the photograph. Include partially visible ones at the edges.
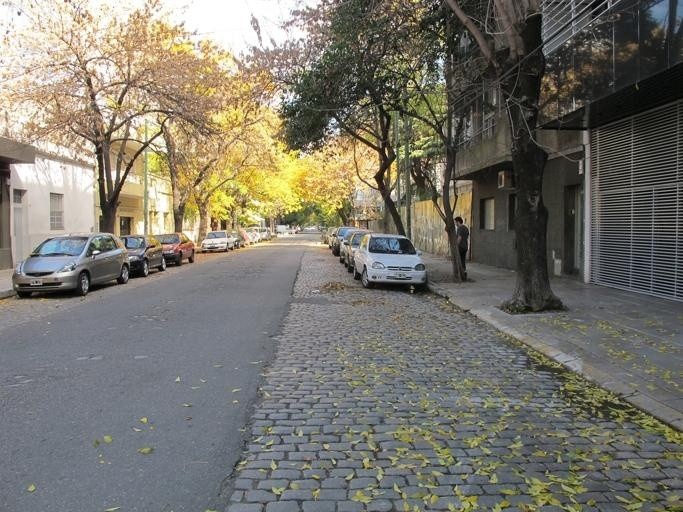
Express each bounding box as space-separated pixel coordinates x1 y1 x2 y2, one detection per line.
497 170 512 191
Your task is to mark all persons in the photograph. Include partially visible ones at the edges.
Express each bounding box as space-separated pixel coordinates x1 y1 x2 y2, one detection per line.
455 217 469 273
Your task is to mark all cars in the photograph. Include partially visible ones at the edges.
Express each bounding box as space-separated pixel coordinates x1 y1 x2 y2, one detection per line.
119 235 166 278
200 222 319 252
319 223 427 288
155 232 196 268
11 231 133 297
